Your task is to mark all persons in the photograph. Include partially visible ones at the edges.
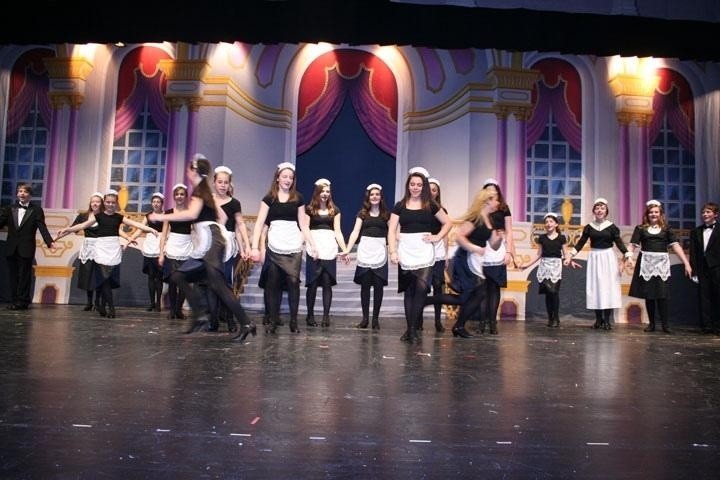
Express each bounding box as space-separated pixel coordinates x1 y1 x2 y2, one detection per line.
619 198 693 334
423 189 506 339
54 153 257 343
418 177 449 332
520 212 582 329
563 198 634 330
0 181 59 310
689 202 720 336
477 178 513 336
387 167 454 344
251 162 390 336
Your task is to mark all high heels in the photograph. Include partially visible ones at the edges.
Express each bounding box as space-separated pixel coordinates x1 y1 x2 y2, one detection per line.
592 320 610 329
147 302 256 342
83 304 115 318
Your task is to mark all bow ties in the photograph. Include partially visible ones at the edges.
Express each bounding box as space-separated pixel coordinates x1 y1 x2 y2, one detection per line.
702 224 713 229
17 204 27 210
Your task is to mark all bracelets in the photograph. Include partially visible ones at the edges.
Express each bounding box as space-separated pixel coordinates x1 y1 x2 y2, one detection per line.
506 250 512 255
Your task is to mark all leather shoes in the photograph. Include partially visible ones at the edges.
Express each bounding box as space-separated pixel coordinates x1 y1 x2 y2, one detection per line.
550 319 559 327
663 324 673 333
7 304 20 310
400 320 423 344
262 316 329 333
644 324 655 331
357 320 380 329
701 328 720 336
436 320 497 338
